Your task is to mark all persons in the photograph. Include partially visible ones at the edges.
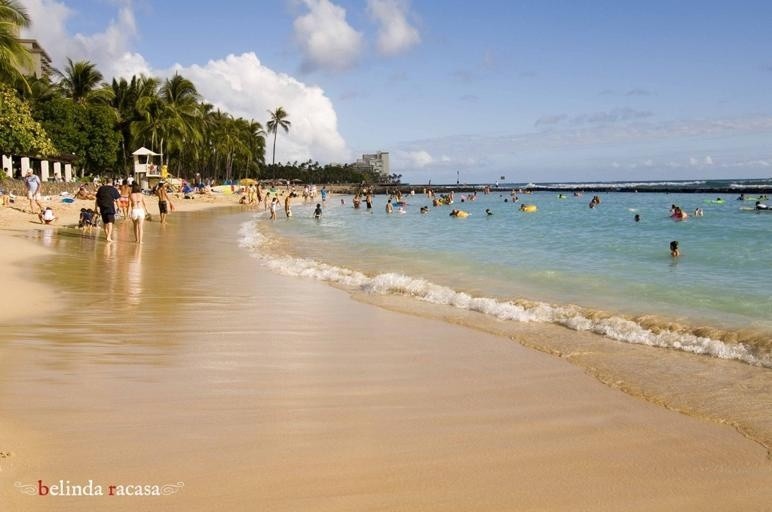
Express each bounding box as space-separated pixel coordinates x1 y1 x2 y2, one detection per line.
629 191 771 257
1 168 600 241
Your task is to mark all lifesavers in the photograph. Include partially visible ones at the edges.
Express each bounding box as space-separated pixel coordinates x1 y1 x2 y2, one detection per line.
702 192 772 214
